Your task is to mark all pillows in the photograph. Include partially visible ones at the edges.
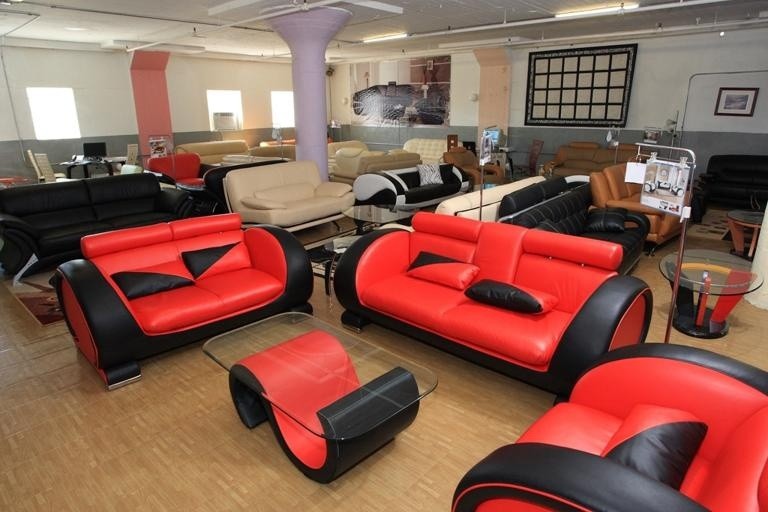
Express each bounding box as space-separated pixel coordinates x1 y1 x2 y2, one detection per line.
601 400 709 492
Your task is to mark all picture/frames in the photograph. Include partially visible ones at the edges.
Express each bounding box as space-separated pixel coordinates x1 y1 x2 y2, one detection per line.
714 87 760 118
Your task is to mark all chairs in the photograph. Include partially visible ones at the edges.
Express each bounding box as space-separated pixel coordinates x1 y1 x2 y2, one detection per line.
452 341 768 512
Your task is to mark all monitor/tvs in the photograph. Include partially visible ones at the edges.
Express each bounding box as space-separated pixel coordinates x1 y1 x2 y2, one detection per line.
83 142 107 161
